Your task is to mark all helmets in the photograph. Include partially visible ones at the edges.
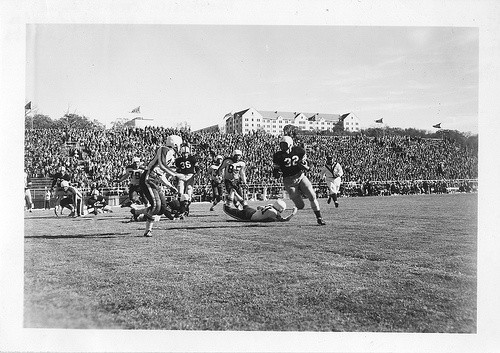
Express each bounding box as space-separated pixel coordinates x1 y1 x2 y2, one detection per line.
181 146 190 154
233 150 242 156
280 136 293 149
166 135 182 149
274 200 286 213
216 155 223 161
326 155 333 164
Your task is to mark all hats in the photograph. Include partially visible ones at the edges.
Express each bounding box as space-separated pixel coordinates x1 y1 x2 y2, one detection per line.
132 157 140 164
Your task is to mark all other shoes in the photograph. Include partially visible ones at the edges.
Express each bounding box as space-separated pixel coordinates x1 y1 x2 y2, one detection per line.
334 200 339 208
317 217 326 225
144 230 153 237
131 208 138 221
327 197 331 204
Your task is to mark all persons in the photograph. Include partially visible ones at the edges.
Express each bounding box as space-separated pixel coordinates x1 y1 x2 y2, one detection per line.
272 135 326 226
222 188 297 221
24 126 478 217
131 134 186 237
